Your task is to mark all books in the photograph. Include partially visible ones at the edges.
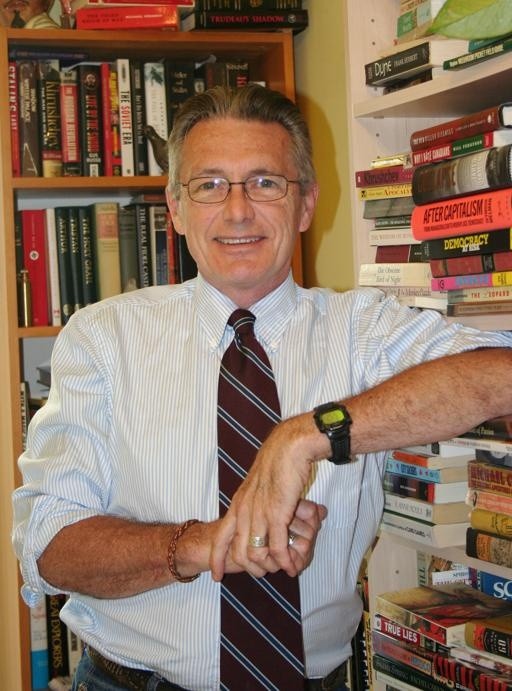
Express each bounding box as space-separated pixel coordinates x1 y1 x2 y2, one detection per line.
66 0 195 31
379 442 476 548
29 593 82 691
166 56 267 118
465 448 512 568
20 360 52 451
353 545 512 691
8 48 169 176
355 102 512 332
15 192 197 327
364 0 512 95
182 0 309 32
460 418 512 441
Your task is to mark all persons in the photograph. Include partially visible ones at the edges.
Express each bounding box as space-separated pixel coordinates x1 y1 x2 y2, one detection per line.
10 84 512 691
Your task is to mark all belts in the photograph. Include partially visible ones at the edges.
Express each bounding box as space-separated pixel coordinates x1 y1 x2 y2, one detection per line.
85 644 348 691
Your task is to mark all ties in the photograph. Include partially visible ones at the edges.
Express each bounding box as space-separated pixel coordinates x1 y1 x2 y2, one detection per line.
218 309 304 691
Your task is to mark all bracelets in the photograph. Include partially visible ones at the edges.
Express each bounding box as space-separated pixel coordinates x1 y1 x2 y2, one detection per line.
167 519 202 583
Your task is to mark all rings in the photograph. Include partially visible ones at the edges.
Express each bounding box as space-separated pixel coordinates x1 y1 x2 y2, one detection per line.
287 533 296 545
249 537 270 547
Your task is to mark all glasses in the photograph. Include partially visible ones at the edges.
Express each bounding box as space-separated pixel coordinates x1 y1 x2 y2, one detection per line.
179 175 300 203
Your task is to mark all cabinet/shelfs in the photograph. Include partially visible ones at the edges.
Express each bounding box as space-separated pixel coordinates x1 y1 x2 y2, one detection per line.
345 1 512 690
0 27 308 691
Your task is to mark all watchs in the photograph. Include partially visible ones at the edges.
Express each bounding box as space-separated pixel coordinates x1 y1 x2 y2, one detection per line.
313 402 351 464
